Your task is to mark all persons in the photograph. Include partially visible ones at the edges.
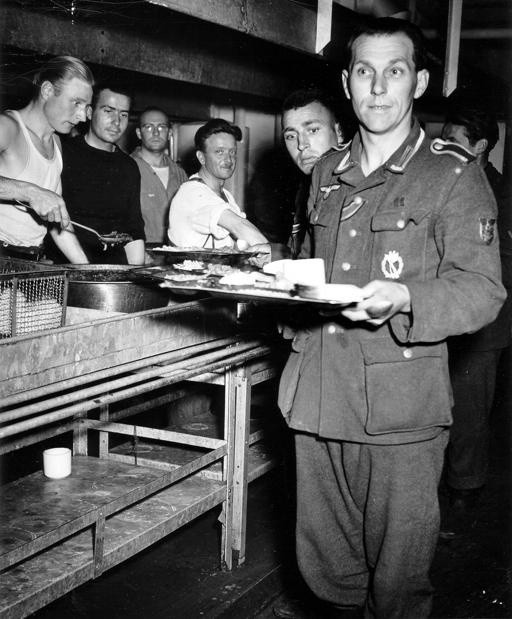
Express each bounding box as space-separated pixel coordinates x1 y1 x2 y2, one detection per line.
279 89 355 255
278 15 506 616
58 82 147 452
129 107 194 428
0 54 95 481
166 118 269 425
437 108 512 542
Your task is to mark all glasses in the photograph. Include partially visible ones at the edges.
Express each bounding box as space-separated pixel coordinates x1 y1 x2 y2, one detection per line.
142 123 169 132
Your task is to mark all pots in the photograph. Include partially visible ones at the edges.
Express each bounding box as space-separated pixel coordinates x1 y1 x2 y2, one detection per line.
47 261 172 313
146 247 257 270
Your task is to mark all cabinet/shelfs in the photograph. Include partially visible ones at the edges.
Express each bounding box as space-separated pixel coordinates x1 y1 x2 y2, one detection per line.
74 297 301 573
3 304 262 617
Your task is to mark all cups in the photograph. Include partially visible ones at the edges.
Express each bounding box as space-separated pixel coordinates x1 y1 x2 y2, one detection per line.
42 447 73 480
